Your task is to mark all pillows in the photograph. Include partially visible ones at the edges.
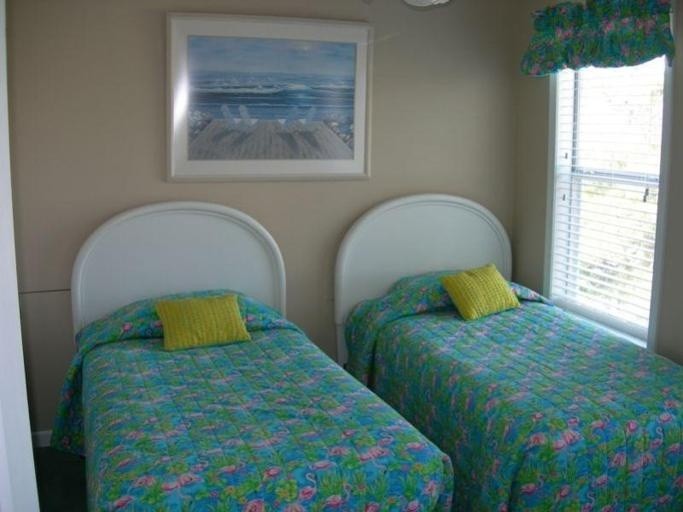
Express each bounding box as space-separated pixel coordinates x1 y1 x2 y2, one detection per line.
156 294 251 351
441 262 520 321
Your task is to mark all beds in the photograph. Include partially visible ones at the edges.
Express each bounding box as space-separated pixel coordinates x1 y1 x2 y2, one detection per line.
331 193 683 512
71 200 453 511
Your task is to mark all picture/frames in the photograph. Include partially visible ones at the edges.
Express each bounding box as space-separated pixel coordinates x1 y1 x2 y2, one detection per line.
165 11 374 181
